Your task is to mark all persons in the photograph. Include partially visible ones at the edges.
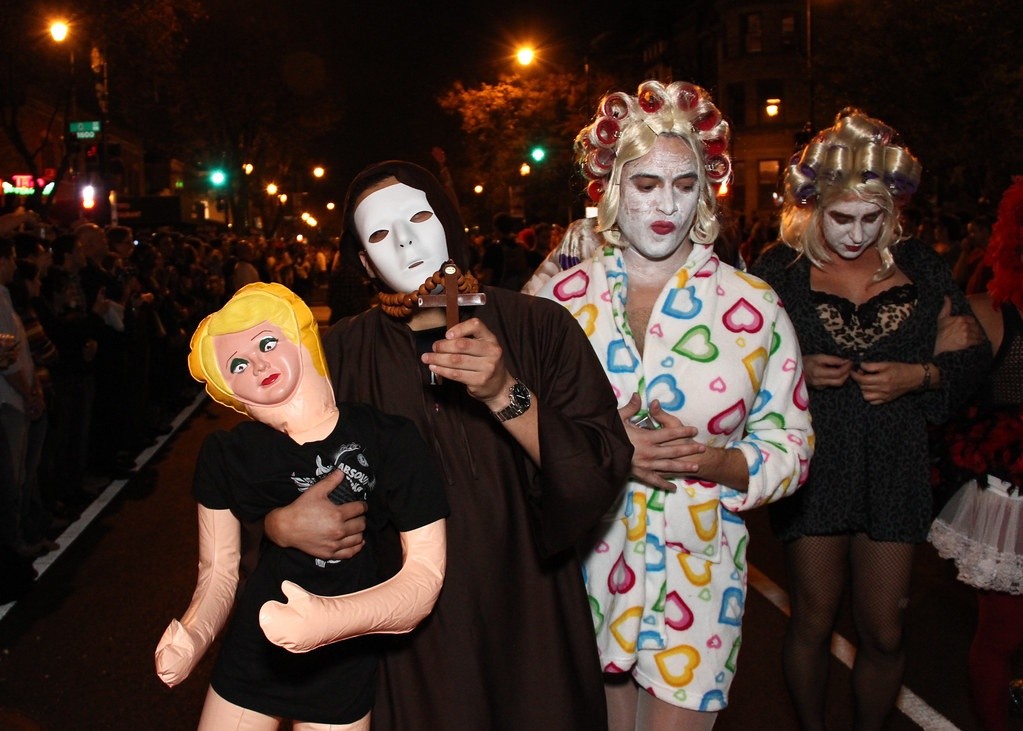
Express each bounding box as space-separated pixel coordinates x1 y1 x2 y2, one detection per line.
458 210 571 290
151 281 454 730
919 171 1023 731
521 81 818 731
1 194 343 570
746 106 996 731
262 157 638 731
707 197 999 295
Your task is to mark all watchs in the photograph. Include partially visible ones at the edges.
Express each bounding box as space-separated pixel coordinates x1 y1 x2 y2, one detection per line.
491 378 533 423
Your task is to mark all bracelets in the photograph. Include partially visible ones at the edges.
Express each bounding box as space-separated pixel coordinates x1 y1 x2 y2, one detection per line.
914 360 933 392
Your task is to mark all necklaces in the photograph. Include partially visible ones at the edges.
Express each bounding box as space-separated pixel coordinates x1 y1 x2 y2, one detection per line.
372 259 478 318
